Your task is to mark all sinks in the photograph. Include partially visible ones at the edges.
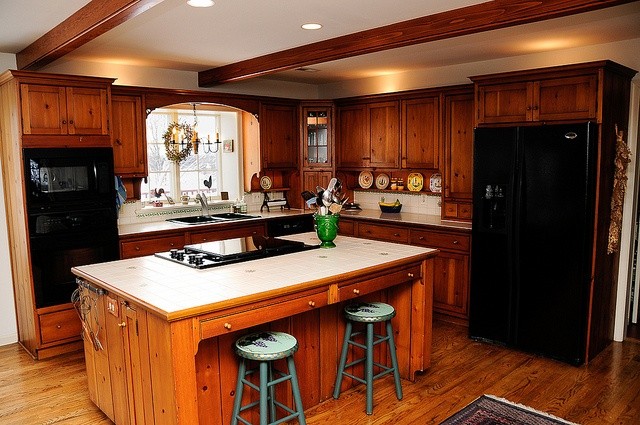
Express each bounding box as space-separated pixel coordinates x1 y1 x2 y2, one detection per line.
212 213 262 221
169 217 224 224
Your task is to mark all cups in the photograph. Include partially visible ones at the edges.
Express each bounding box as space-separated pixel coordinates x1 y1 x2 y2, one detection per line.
179 193 191 205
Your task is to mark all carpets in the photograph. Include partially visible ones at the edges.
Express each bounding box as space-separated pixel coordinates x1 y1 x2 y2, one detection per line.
439 394 579 425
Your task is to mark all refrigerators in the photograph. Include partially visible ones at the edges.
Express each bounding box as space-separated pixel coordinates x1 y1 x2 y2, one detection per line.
465 120 600 369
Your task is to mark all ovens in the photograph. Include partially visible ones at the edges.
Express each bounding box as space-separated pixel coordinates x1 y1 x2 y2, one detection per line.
21 146 122 309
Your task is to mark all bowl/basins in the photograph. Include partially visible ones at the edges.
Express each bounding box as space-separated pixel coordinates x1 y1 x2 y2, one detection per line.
389 177 397 192
396 178 404 192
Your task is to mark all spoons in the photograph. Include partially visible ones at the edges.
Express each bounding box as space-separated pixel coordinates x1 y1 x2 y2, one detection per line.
322 189 334 207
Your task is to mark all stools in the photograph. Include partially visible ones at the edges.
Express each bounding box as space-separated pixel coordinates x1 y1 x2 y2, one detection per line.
333 303 403 414
232 331 307 425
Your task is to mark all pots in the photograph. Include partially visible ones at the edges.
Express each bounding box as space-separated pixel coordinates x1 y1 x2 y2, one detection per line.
378 198 402 214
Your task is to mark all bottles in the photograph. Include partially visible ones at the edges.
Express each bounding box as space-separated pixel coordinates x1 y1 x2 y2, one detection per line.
240 196 248 213
229 204 233 213
233 198 241 213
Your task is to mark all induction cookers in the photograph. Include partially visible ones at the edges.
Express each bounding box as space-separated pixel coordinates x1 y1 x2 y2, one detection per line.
170 234 304 266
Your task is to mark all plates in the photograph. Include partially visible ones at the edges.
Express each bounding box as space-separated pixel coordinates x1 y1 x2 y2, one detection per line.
259 176 273 189
406 172 424 192
375 173 390 190
429 173 442 194
357 169 374 189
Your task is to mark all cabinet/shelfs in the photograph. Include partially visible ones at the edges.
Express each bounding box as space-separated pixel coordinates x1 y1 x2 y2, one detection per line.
113 95 143 174
402 96 439 169
17 303 83 362
446 95 475 201
468 59 637 126
261 105 295 166
188 225 265 243
357 224 407 243
0 73 114 147
341 101 400 169
122 233 185 257
338 221 353 235
410 230 470 318
304 171 333 211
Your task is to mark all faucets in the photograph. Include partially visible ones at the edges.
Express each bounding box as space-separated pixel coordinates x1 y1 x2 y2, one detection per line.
196 193 211 219
